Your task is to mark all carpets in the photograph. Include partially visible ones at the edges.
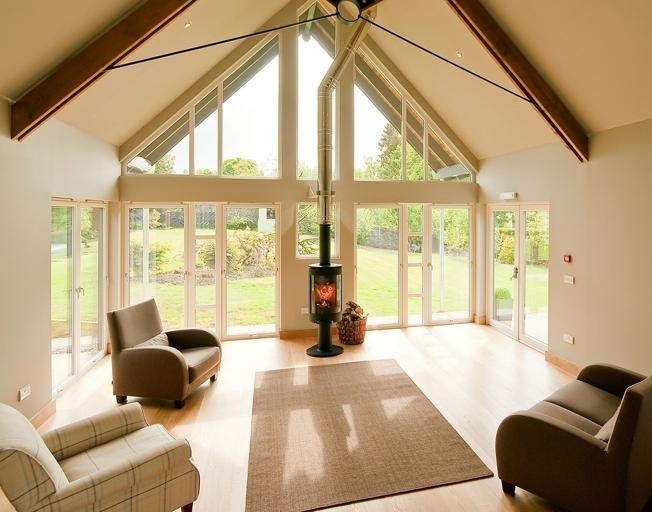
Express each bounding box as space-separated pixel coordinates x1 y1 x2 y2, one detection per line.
245 359 495 511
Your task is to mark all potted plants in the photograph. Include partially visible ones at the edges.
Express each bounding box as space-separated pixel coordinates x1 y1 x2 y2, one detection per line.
493 287 513 321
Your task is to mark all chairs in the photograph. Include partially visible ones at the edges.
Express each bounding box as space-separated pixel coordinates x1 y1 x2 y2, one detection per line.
0 403 201 512
495 363 652 512
105 298 222 409
